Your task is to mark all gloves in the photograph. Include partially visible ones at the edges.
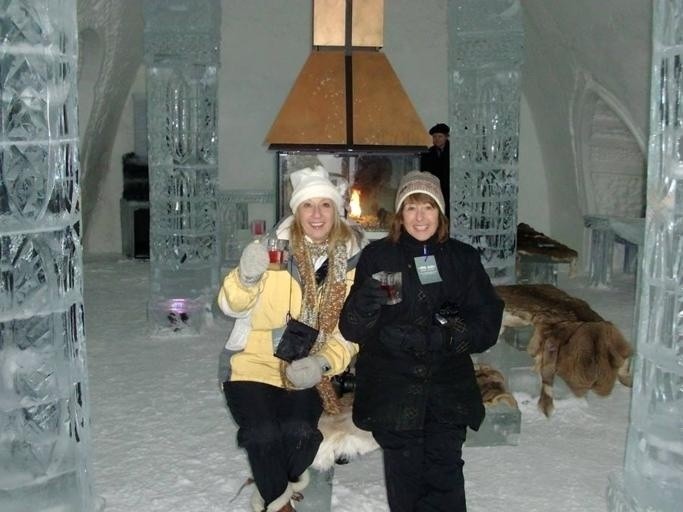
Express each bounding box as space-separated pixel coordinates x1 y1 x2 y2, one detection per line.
285 355 331 389
394 322 443 357
352 276 387 313
239 240 270 288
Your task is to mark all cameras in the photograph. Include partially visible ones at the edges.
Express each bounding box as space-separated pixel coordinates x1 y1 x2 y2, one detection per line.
435 313 448 328
330 371 356 398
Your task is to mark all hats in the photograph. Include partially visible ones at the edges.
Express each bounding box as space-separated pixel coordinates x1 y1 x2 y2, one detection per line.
395 169 446 216
429 123 450 137
289 166 341 215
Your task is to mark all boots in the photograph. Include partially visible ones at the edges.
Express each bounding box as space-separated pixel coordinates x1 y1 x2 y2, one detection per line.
251 470 310 512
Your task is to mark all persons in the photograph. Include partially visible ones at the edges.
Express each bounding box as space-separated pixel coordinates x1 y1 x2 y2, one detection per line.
419 124 450 224
338 170 504 512
216 163 368 512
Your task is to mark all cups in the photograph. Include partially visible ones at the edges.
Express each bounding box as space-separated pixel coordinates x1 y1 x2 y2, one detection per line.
264 239 287 271
373 271 402 306
